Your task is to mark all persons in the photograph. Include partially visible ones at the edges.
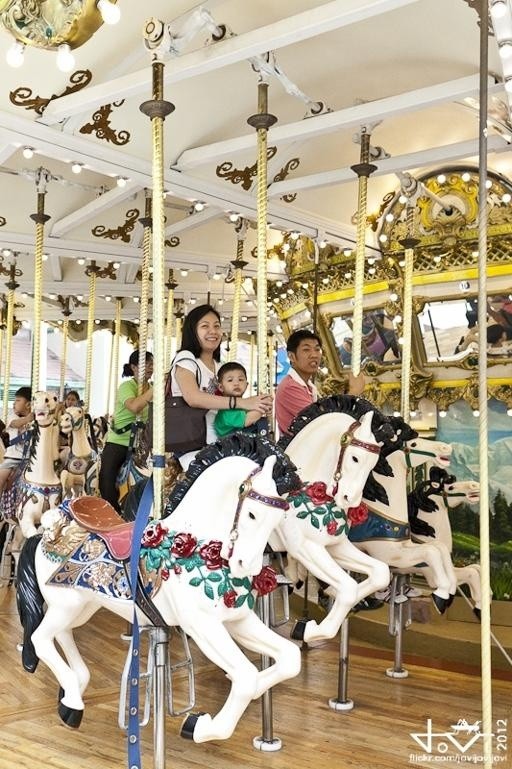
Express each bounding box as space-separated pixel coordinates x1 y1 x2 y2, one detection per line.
276 330 365 434
168 305 274 473
99 350 153 516
0 387 79 502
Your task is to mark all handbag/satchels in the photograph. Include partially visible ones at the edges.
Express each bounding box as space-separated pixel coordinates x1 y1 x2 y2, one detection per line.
148 355 211 453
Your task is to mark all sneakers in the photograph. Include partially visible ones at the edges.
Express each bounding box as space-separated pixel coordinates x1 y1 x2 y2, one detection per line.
317 586 359 615
375 588 409 604
354 595 384 612
401 585 423 598
274 573 293 584
